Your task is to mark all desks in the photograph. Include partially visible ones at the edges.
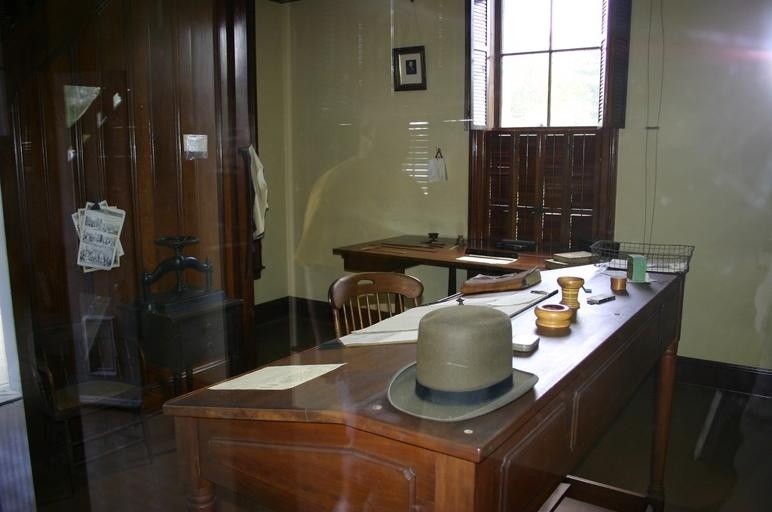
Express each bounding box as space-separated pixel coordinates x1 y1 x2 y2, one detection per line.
161 233 690 512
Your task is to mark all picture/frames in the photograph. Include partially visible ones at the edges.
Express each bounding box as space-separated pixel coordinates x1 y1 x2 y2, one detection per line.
391 45 427 91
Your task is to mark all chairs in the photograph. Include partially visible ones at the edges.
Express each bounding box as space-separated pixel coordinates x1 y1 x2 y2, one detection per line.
19 322 154 500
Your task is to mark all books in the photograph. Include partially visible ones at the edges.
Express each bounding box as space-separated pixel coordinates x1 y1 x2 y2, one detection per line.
552 250 601 263
606 256 689 274
460 264 542 294
543 257 601 270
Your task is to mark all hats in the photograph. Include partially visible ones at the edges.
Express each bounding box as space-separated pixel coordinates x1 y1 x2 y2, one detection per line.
384 301 539 423
